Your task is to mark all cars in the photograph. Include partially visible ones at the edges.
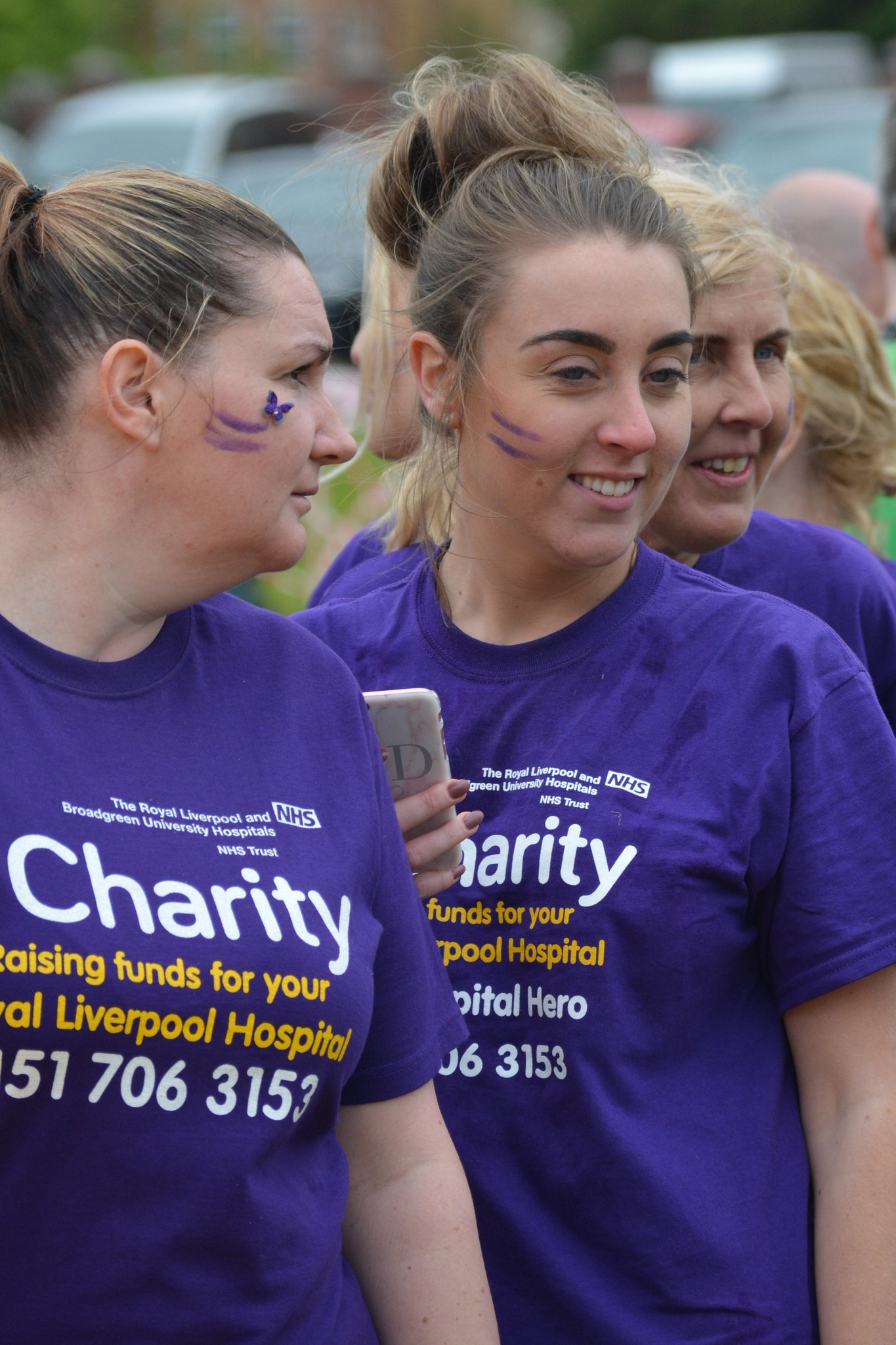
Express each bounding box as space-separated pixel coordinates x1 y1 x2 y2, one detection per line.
24 72 386 313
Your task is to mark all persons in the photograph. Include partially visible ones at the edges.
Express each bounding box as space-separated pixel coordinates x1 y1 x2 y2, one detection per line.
309 146 896 734
0 163 501 1345
278 48 896 1345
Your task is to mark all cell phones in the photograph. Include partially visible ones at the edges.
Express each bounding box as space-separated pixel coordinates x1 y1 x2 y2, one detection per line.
364 688 463 872
